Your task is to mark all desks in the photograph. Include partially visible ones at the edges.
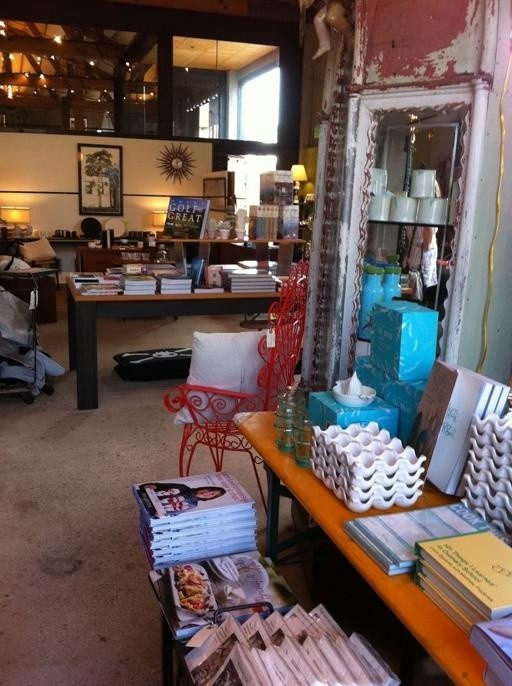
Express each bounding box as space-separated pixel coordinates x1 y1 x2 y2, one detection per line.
232 410 511 685
65 271 306 410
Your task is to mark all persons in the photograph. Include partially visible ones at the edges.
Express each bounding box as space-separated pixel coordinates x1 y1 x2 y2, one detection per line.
404 178 441 311
141 480 226 521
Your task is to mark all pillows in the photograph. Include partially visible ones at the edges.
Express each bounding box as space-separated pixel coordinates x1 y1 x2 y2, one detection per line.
173 323 297 426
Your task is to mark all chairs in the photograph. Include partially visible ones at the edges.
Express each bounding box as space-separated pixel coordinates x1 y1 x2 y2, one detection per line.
163 258 309 517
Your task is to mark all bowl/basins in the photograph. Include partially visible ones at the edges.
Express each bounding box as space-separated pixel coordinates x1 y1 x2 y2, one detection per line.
333 384 377 407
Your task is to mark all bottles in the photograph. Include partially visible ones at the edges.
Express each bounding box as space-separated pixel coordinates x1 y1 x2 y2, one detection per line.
358 254 402 341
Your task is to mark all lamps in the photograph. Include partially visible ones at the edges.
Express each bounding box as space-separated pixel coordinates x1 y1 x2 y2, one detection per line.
290 164 308 203
152 211 169 227
1 206 31 228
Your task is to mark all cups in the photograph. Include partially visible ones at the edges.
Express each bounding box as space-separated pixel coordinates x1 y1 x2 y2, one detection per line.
273 386 320 468
368 168 448 225
206 229 245 240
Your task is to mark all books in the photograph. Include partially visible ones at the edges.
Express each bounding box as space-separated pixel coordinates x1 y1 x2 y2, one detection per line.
469 617 511 680
157 196 210 240
344 502 496 576
130 471 259 567
413 532 510 639
405 361 510 497
71 259 297 297
147 551 300 638
183 606 402 683
248 170 300 240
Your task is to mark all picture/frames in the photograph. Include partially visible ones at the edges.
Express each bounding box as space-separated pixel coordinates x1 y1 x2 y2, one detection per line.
76 142 124 217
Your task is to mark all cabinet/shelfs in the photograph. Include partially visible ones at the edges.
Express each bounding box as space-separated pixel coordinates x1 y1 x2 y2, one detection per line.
326 81 488 390
